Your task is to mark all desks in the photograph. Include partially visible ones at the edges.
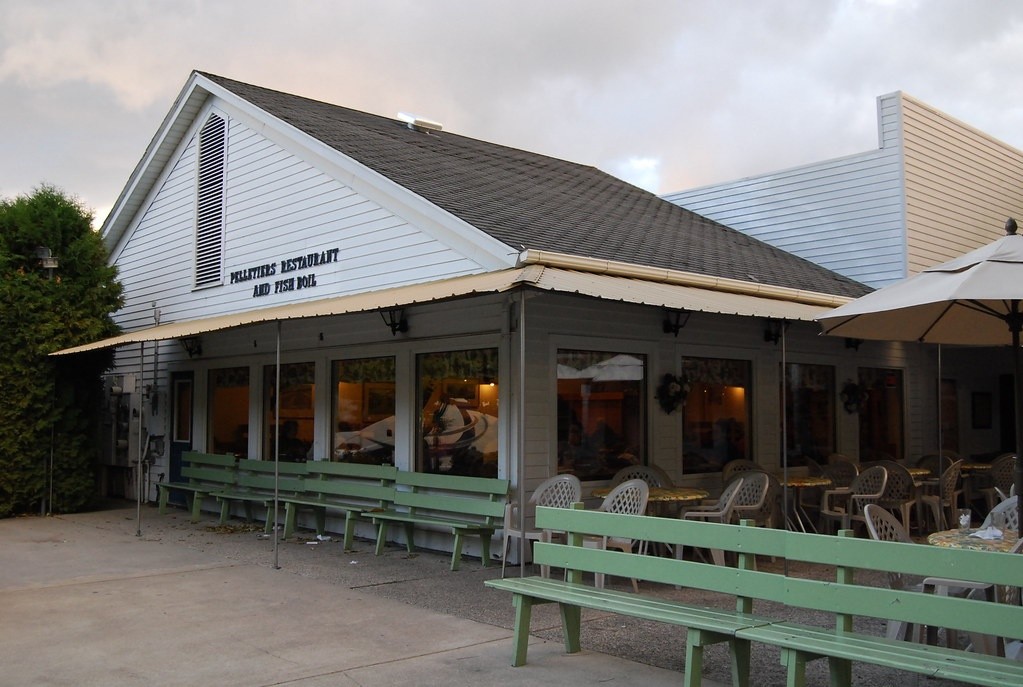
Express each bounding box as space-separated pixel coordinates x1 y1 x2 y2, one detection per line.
770 476 831 535
961 464 993 475
927 527 1020 552
908 467 932 480
590 486 711 581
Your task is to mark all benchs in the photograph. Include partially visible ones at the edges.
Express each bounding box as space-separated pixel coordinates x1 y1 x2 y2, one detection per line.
488 504 782 687
361 470 511 571
733 529 1023 687
154 451 236 522
210 458 308 535
274 460 399 550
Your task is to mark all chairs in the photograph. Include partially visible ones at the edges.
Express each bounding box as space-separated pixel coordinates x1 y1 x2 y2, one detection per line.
864 503 970 641
808 456 827 477
823 466 888 535
869 462 923 538
978 495 1018 528
821 463 860 488
500 474 581 578
610 464 669 488
912 536 1023 687
675 477 745 590
922 458 964 531
975 454 1016 513
943 456 954 472
724 473 769 571
718 459 759 485
583 479 649 594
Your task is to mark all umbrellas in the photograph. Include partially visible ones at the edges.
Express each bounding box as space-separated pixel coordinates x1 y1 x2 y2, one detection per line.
815 215 1023 541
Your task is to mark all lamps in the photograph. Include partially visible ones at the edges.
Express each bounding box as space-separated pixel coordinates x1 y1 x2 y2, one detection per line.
763 318 792 345
177 336 202 359
379 308 408 337
661 307 692 339
844 337 865 352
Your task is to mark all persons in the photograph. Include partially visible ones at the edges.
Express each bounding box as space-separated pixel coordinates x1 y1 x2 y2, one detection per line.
451 448 478 476
270 420 303 449
338 422 353 450
226 424 248 456
709 420 745 465
558 394 583 457
591 423 639 469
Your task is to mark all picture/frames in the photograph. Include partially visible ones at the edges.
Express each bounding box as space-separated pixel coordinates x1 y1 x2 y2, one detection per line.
360 381 396 422
274 385 316 419
441 378 480 408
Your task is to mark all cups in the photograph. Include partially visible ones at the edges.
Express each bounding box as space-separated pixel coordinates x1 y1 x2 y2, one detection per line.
956 508 971 534
